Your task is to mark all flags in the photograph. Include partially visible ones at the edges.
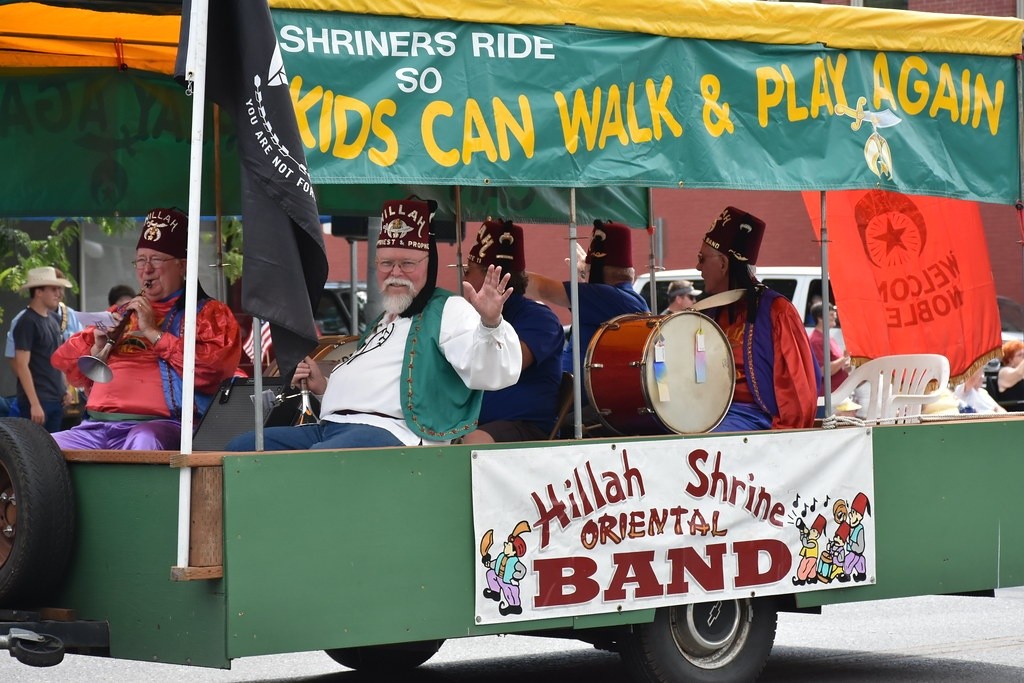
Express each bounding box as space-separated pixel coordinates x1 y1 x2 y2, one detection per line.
172 0 328 389
242 318 273 364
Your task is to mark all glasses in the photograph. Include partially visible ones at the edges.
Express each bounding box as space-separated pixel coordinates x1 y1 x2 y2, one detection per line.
462 263 490 277
131 257 176 269
697 253 723 264
376 254 430 273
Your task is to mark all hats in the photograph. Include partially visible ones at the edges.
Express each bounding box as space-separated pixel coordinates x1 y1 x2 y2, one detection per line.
702 206 766 324
666 281 703 297
19 267 74 290
379 194 438 319
585 217 634 285
136 208 212 310
468 218 527 275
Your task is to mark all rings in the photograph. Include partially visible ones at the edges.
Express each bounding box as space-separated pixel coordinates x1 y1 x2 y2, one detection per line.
139 304 143 307
496 287 505 294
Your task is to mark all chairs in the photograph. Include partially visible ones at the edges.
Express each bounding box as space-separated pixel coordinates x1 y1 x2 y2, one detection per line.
816 354 952 425
551 372 573 441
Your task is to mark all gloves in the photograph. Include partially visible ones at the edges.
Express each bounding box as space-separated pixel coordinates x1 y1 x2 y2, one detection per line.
566 241 588 273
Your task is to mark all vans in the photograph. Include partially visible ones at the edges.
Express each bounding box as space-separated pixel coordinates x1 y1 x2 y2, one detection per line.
632 266 851 368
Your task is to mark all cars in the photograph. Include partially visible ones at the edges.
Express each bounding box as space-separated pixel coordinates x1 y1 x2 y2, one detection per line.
976 294 1024 411
315 281 369 339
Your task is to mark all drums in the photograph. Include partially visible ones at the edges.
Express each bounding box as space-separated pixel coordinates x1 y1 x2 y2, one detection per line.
261 335 364 377
583 307 737 434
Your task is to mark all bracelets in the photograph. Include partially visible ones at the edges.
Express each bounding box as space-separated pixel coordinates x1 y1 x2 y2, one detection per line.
152 332 163 347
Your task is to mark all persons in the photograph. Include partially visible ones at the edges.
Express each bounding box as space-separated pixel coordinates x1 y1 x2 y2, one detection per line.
2 265 137 433
659 281 703 314
695 206 822 434
950 337 1024 414
806 299 849 396
457 219 566 445
225 200 522 450
524 220 650 428
45 206 245 450
